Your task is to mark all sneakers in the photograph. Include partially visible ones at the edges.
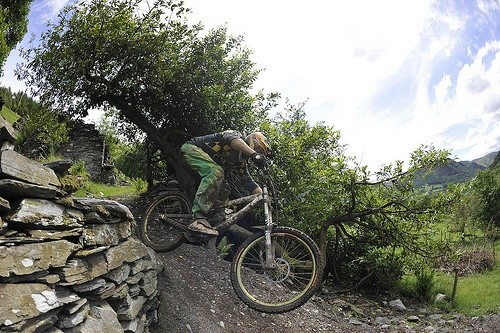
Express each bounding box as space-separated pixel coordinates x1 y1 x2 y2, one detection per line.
188 219 219 238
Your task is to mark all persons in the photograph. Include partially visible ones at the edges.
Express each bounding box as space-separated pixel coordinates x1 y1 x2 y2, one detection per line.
179 128 273 236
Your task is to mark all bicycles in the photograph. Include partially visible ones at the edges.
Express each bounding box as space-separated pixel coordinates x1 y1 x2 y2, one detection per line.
139 154 325 314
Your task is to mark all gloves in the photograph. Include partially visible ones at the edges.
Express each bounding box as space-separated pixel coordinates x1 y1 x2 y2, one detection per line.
250 152 266 166
271 198 283 211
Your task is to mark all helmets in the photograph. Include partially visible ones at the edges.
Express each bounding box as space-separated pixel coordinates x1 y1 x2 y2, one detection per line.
239 132 273 162
